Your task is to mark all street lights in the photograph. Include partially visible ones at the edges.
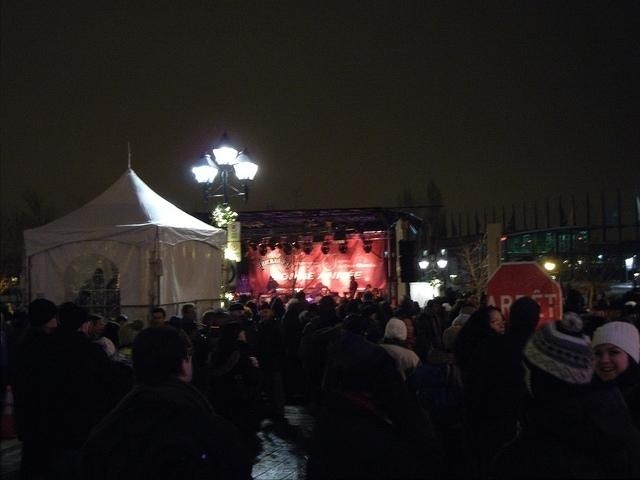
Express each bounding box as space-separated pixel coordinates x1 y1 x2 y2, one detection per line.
190 129 264 306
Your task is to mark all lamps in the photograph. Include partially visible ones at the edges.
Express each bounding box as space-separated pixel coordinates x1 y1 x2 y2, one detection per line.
248 235 373 256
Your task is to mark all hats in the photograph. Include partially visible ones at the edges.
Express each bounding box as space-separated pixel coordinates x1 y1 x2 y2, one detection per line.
591 321 640 364
29 298 57 327
525 313 593 385
132 327 184 384
385 318 407 340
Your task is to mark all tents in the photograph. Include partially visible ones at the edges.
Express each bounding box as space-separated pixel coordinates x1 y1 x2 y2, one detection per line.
22 168 228 331
